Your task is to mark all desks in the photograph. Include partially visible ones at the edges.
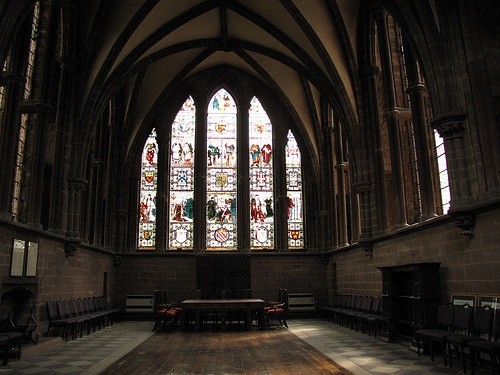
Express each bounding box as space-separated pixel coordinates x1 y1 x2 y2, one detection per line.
182 297 265 332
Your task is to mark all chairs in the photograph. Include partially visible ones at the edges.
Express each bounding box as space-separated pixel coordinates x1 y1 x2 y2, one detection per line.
316 293 384 340
415 299 454 363
446 304 494 370
262 286 289 329
468 309 500 375
430 303 473 366
0 317 23 368
45 294 125 344
151 288 185 333
